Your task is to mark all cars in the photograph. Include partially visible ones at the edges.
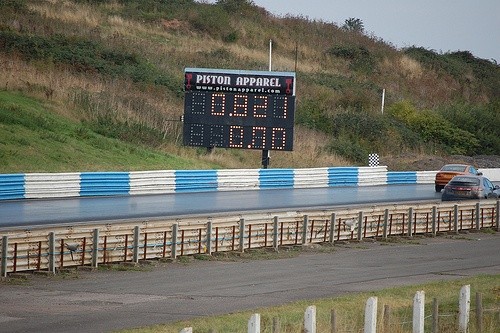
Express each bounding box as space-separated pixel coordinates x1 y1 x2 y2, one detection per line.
441 175 500 202
435 164 483 192
